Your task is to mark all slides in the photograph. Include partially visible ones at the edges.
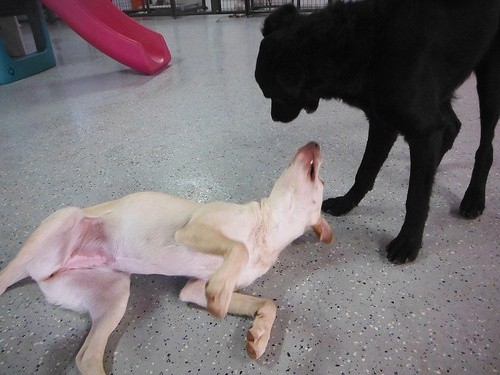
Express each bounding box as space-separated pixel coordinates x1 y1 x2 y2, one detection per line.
41 0 173 76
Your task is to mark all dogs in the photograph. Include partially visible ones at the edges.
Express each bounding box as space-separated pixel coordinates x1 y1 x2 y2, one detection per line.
0 141 333 375
255 0 500 264
